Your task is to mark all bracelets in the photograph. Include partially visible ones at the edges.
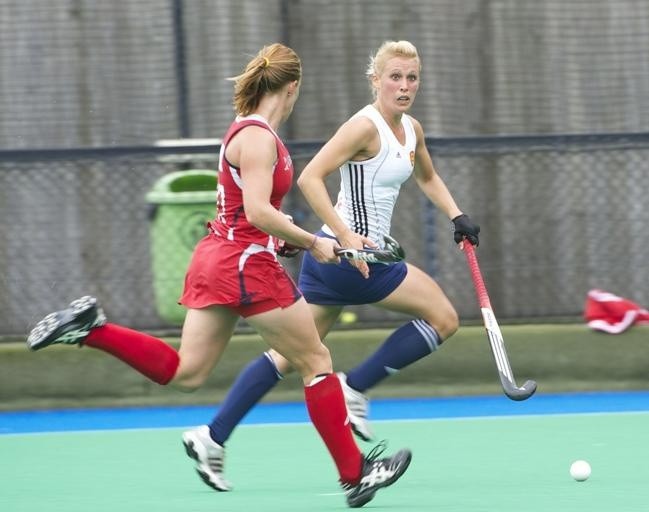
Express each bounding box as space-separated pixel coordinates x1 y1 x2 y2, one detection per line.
309 237 318 250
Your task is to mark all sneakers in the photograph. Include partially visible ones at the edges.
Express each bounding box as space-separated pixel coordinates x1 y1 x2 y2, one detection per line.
27 296 106 349
339 449 411 507
336 372 373 441
183 425 230 490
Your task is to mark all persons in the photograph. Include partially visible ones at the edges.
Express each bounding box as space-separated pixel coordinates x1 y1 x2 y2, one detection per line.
183 40 481 492
27 42 413 509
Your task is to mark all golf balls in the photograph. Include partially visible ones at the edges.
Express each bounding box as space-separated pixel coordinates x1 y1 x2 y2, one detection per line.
571 460 591 481
341 312 356 324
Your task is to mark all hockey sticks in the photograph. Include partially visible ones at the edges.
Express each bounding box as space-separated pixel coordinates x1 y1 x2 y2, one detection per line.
274 235 405 263
463 239 537 401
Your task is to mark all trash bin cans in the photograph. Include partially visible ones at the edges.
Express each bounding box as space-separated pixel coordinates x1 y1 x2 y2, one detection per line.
147 170 221 326
